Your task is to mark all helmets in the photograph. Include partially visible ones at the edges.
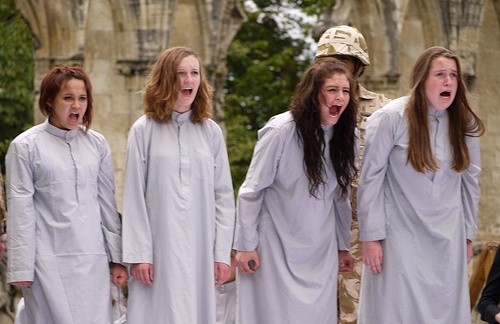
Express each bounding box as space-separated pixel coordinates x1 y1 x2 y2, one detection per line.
315 25 372 65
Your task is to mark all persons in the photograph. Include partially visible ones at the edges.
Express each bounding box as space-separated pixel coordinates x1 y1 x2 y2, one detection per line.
231 58 361 324
314 25 392 324
6 65 128 324
357 46 484 324
122 47 235 324
